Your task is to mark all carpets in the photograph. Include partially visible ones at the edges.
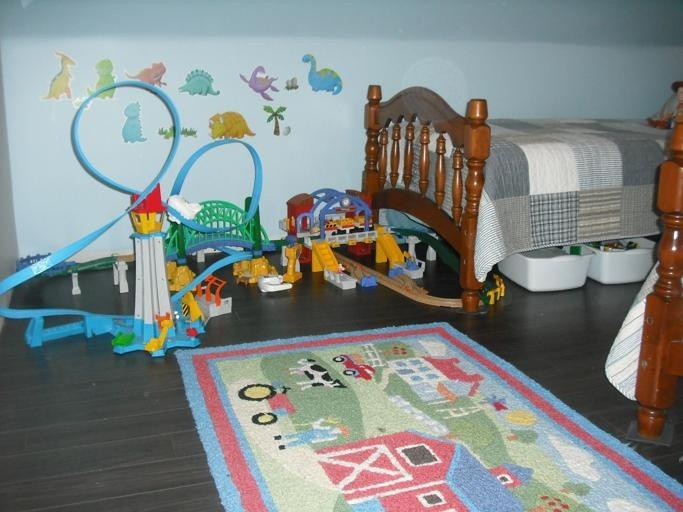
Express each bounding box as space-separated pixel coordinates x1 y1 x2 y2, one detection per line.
173 322 683 512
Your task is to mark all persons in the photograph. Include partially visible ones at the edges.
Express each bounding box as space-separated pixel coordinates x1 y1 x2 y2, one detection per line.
646 81 683 129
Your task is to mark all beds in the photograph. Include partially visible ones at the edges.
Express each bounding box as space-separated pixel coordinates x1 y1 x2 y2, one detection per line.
604 103 683 440
360 84 671 313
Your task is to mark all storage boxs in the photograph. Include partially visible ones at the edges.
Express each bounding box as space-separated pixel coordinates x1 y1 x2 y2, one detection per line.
583 238 657 284
498 243 596 292
